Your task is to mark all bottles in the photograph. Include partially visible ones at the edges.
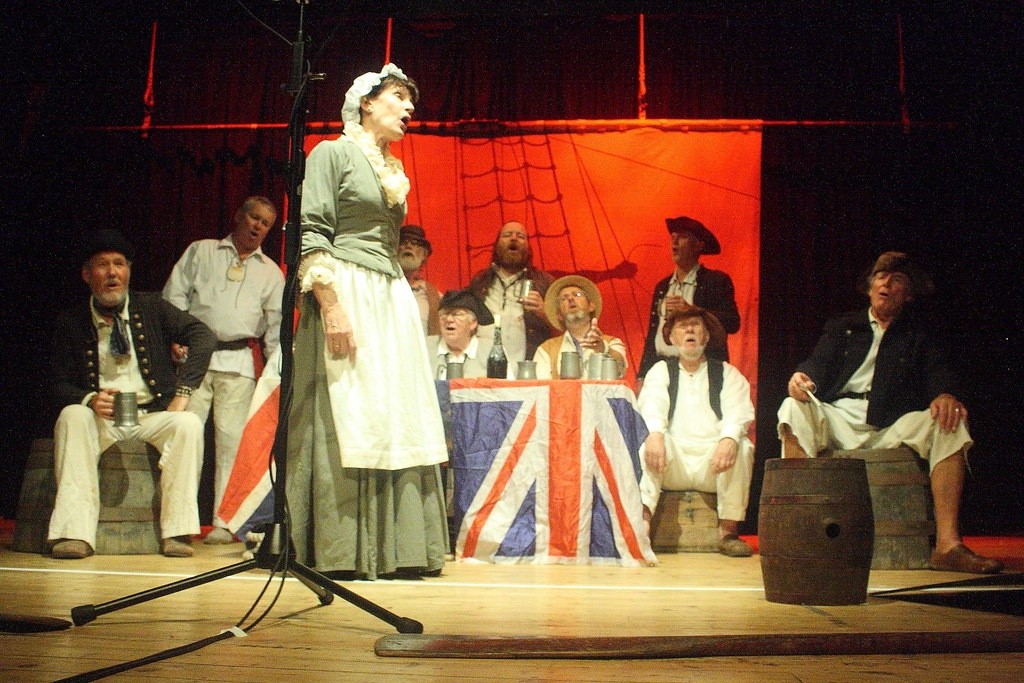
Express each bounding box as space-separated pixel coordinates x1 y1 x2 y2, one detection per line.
487 326 508 378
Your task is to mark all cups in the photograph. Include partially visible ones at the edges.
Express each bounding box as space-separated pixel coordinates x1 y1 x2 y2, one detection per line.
445 362 464 380
513 278 533 302
516 350 622 381
113 391 141 427
660 296 681 320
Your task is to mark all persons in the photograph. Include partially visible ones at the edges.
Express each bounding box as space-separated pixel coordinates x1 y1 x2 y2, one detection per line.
397 224 446 382
423 289 500 378
468 221 558 382
532 284 629 380
636 216 740 400
44 229 219 559
282 63 452 581
159 196 288 543
775 251 1006 574
636 305 755 556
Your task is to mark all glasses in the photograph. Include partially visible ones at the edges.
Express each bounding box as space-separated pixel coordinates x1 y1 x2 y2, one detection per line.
400 238 422 244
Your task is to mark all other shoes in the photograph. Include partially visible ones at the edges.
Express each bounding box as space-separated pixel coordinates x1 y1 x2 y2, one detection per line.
717 533 751 557
204 529 235 544
930 542 1004 573
52 538 93 559
163 536 195 558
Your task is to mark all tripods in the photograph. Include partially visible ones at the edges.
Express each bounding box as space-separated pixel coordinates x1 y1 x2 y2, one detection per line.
72 1 426 635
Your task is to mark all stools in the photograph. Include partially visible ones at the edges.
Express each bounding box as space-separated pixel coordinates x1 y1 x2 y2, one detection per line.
649 490 720 552
11 437 163 554
831 446 935 570
757 457 875 607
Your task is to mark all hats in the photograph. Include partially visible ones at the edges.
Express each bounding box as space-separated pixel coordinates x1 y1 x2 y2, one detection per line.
665 215 720 255
399 224 432 256
437 288 494 326
543 276 602 332
341 63 408 125
662 307 725 349
870 252 915 279
75 229 134 269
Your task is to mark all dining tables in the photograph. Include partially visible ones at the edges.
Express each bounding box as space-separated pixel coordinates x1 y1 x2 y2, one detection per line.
247 374 636 566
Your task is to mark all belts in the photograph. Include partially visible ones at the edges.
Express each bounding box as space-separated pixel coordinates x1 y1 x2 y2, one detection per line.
834 391 872 400
175 337 248 350
